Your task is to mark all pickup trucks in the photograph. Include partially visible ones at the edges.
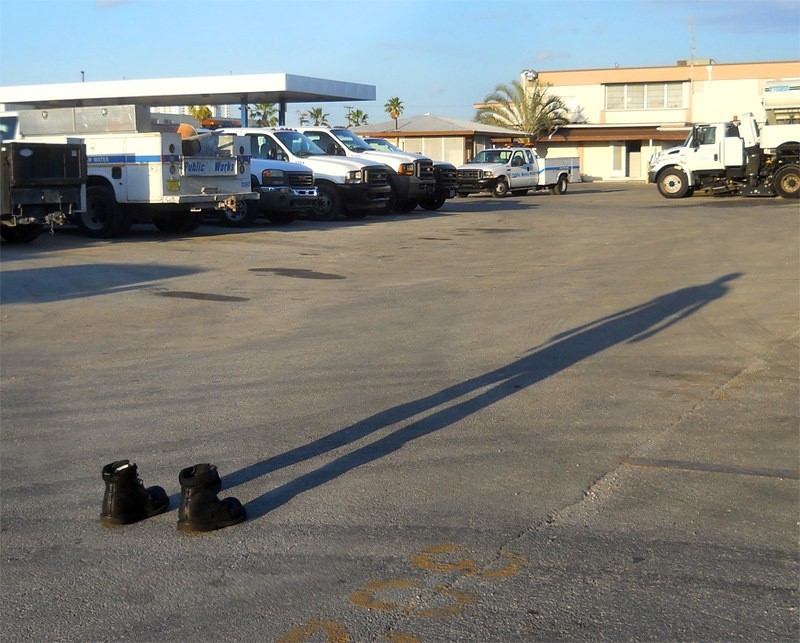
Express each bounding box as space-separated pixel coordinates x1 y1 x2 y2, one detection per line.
0 102 459 242
456 146 582 197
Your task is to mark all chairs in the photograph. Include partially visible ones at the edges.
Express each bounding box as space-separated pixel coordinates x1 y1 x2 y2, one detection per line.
259 143 270 159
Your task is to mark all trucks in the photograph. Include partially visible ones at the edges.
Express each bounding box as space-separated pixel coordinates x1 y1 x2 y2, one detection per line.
647 112 800 201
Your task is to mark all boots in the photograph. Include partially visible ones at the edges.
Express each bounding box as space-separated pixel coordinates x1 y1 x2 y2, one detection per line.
176 464 247 530
100 460 170 525
